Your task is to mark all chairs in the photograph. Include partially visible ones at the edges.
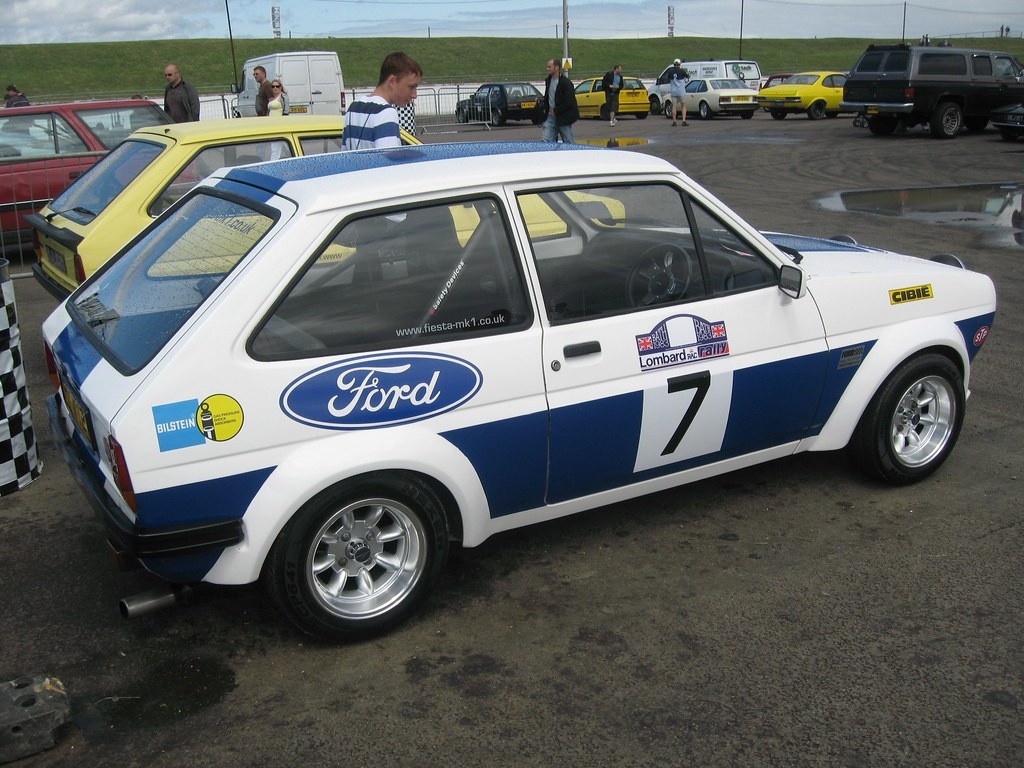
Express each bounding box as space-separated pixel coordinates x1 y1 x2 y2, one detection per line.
626 83 634 89
721 82 730 88
512 89 522 99
404 206 464 277
597 85 604 91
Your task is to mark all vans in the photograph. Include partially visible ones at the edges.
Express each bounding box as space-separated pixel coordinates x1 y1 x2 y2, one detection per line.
230 52 346 117
646 60 762 116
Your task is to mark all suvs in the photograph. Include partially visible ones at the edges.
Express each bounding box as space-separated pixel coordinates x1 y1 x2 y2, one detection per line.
839 43 1024 140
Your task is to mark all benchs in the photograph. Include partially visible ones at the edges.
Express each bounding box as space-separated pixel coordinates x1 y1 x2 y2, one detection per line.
195 278 328 356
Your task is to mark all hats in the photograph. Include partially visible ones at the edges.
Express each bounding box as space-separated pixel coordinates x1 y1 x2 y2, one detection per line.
674 59 681 65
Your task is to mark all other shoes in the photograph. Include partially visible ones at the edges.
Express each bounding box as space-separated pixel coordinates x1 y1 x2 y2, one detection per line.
613 118 617 123
682 122 689 126
610 121 615 126
672 122 676 126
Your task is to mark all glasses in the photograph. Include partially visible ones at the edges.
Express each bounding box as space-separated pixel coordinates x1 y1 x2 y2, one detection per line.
272 85 281 88
253 73 263 76
165 72 177 77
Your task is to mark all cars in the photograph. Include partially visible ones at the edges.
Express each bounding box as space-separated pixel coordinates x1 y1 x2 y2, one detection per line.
41 140 999 646
21 114 625 306
758 73 794 112
988 102 1024 142
0 98 214 246
455 82 544 127
662 77 761 120
756 71 850 121
573 77 650 122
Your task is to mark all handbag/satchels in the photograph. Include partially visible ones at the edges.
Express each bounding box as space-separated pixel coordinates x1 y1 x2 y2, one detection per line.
532 96 547 127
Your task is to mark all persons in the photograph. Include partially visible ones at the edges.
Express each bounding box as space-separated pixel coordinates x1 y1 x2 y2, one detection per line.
162 63 202 122
268 79 289 116
252 66 274 116
541 58 578 144
3 84 30 108
602 64 623 127
341 52 422 148
667 58 691 126
